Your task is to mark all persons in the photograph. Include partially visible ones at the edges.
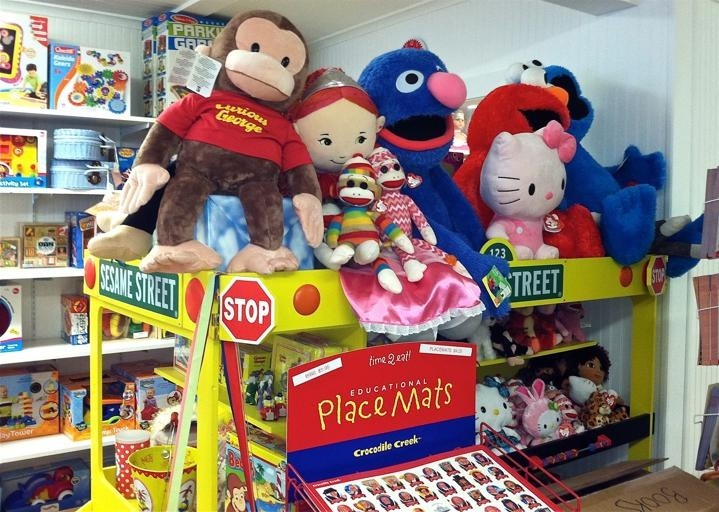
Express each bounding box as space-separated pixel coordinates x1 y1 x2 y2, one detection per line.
449 109 471 156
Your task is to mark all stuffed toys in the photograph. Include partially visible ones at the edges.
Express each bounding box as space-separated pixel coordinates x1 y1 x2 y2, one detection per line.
449 83 607 260
324 152 415 300
291 67 486 337
473 121 577 263
499 57 706 277
363 144 460 285
440 303 586 361
140 8 324 274
356 36 510 319
472 346 628 451
86 161 177 262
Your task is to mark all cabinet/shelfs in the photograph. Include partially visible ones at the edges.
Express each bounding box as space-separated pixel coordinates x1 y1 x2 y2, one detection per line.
84 249 667 512
0 103 175 512
